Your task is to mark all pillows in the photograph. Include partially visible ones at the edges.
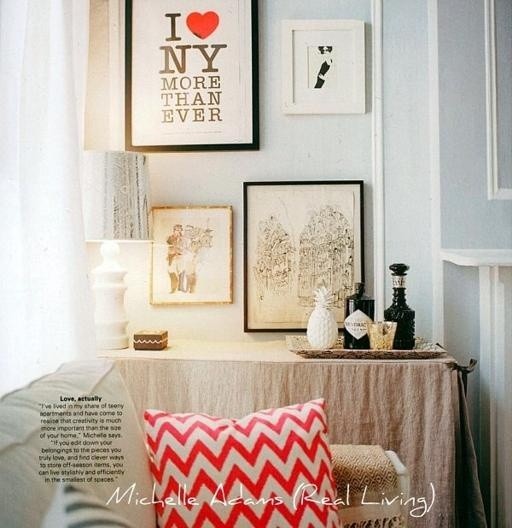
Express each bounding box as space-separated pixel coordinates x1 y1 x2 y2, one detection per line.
141 396 341 527
41 482 139 527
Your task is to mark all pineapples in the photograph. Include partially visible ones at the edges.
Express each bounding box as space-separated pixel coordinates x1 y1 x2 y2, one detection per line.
307 285 338 350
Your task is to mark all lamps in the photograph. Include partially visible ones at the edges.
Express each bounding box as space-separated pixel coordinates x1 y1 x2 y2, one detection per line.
76 150 154 353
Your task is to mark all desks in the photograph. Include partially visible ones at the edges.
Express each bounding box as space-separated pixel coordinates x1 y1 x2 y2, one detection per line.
110 339 457 526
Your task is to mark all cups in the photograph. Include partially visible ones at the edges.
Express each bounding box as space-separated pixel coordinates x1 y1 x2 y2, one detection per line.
366 319 401 349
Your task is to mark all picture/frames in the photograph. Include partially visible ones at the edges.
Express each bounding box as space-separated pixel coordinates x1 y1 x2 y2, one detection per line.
149 203 235 308
124 0 260 154
240 179 366 333
278 19 369 116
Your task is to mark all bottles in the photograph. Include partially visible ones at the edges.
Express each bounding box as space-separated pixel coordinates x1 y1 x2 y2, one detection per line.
384 262 417 350
342 280 375 347
